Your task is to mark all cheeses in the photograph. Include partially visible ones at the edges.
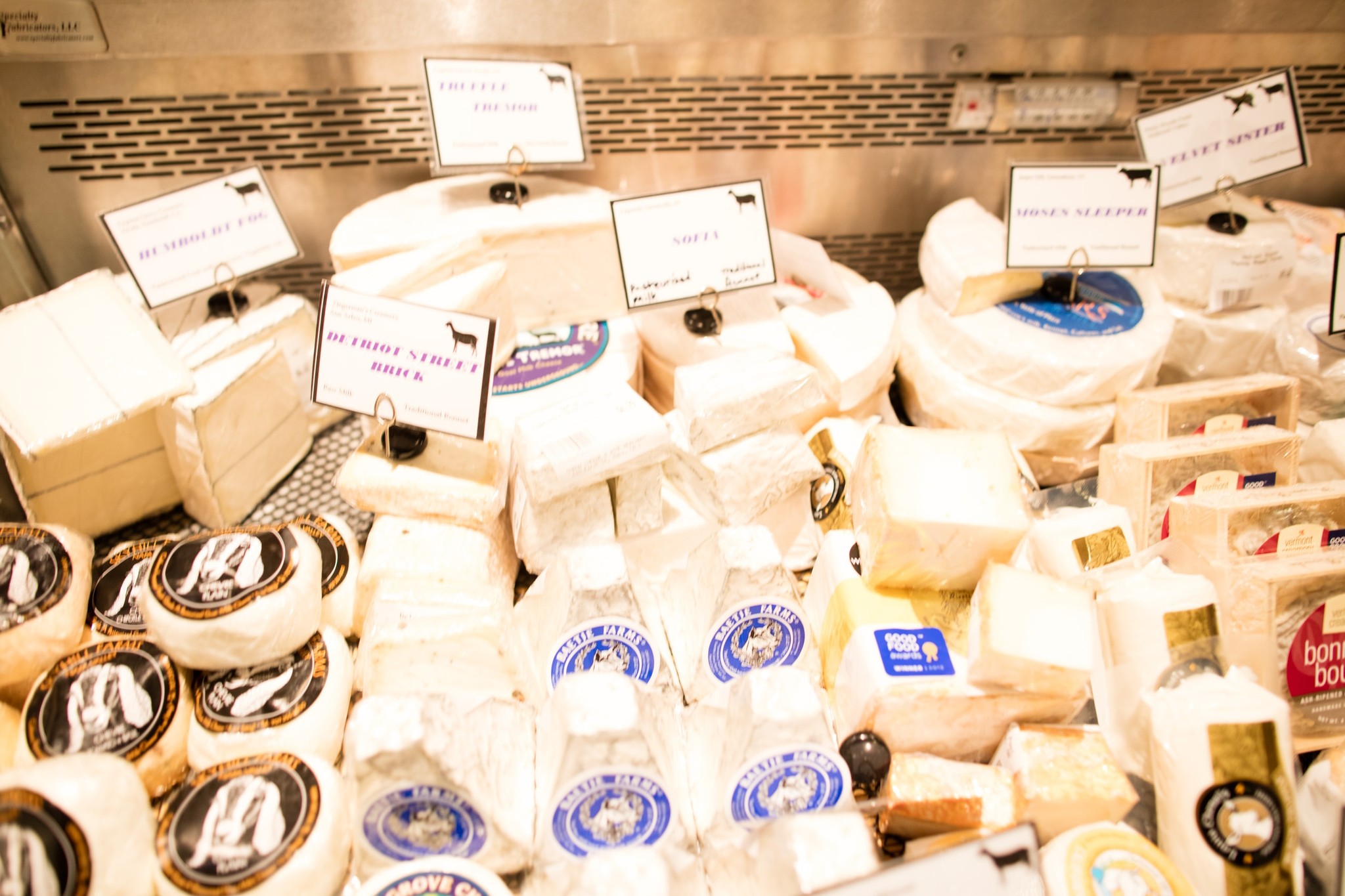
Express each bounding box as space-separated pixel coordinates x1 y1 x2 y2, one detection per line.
0 171 1345 896
